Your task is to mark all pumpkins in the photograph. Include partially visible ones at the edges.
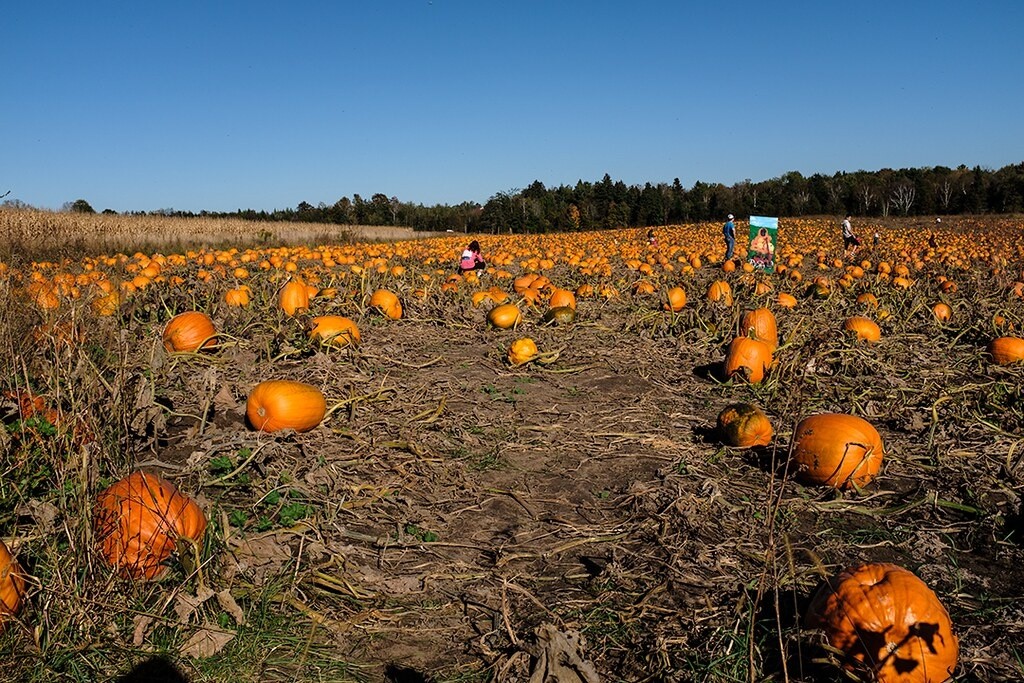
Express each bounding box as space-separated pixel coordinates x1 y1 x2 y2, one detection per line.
0 539 26 635
90 471 205 583
247 380 328 435
790 414 884 489
717 403 773 447
0 220 1024 384
803 562 958 683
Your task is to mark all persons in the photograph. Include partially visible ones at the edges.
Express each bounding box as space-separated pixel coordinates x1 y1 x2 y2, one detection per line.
935 218 941 224
647 230 655 246
842 215 879 256
722 214 736 260
457 241 486 278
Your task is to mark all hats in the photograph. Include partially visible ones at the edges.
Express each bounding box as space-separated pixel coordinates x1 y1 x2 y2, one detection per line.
728 214 734 220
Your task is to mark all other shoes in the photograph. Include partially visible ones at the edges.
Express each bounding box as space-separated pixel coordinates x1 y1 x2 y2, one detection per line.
850 252 854 256
477 273 481 279
